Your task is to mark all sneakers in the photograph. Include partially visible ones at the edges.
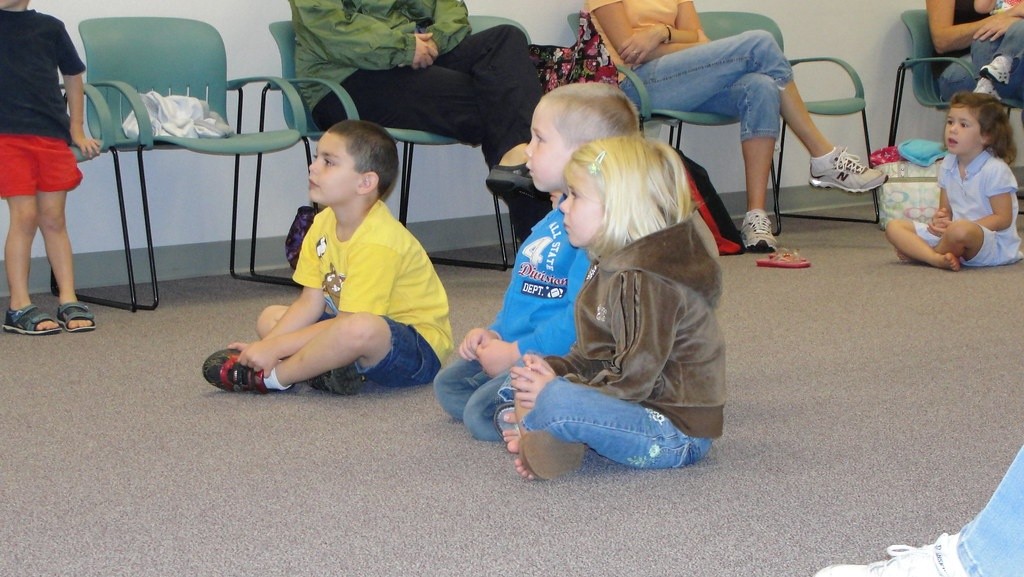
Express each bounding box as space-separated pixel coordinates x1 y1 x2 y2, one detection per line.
202 348 296 395
310 366 362 396
979 58 1010 86
740 209 778 253
814 533 967 577
972 78 1002 100
809 146 889 193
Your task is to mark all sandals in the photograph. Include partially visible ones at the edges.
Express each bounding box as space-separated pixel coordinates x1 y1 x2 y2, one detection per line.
56 302 95 332
3 304 62 335
522 433 586 481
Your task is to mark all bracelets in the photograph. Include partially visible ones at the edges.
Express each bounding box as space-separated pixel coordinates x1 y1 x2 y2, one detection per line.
665 23 672 44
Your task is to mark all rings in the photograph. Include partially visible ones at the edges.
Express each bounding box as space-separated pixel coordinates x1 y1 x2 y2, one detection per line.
633 50 638 57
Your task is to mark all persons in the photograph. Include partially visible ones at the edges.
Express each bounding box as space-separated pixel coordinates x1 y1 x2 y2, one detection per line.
493 135 726 483
882 88 1023 271
806 443 1024 577
433 82 640 441
200 118 456 398
0 0 103 336
587 0 891 251
288 0 548 243
925 0 1023 101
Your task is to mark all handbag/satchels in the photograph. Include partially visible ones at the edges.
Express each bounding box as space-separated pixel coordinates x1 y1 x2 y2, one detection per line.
873 161 940 232
528 9 616 96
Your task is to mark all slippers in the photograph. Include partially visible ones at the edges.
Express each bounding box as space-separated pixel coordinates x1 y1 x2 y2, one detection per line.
485 161 551 215
757 247 811 268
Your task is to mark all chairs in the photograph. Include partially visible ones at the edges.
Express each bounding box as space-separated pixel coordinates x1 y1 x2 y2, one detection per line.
403 17 532 268
696 11 880 224
888 9 1024 214
250 22 508 281
56 84 136 313
78 17 313 310
568 13 781 236
